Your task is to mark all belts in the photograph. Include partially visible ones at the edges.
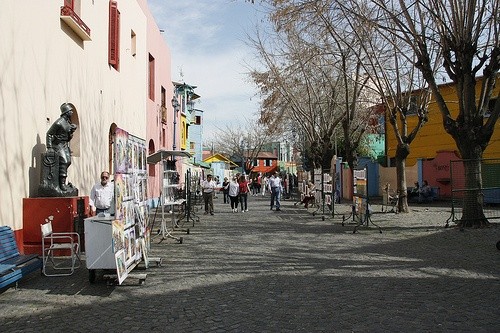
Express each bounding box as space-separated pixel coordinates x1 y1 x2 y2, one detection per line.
97 208 109 210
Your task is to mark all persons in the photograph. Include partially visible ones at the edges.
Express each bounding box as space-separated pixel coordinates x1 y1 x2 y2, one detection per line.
407 180 431 201
88 171 114 216
46 103 77 190
201 171 316 215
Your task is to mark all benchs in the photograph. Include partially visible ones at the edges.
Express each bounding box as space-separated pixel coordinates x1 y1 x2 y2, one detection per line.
0 225 43 289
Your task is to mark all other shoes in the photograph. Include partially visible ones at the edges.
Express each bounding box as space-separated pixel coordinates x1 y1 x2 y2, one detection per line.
244 208 248 211
235 208 238 212
204 211 208 214
270 207 272 210
232 209 235 213
241 209 244 212
276 208 281 211
210 212 214 215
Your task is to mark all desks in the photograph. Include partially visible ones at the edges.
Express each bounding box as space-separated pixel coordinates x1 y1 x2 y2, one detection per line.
83 211 145 284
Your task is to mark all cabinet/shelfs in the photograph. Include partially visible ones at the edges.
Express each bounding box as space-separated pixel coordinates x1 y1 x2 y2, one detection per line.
161 170 189 244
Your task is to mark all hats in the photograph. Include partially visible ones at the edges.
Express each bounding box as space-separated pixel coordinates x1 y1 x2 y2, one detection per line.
224 177 228 180
207 174 212 178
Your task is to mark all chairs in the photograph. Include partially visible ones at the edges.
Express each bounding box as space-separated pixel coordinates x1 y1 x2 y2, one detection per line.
40 221 80 276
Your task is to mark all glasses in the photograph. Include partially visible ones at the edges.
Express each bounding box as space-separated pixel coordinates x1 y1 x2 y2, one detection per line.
102 176 108 179
